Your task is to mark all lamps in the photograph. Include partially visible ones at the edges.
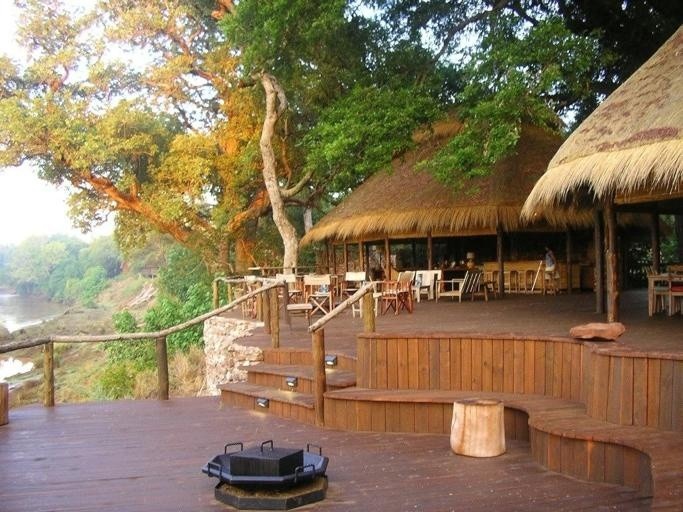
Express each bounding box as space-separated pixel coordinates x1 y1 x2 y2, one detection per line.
324 355 337 369
254 396 269 411
283 376 296 388
466 252 474 265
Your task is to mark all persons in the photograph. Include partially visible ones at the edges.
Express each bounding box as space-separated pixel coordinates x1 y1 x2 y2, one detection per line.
541 243 562 296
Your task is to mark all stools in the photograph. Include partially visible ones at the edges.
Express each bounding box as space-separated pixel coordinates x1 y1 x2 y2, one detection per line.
498 268 561 298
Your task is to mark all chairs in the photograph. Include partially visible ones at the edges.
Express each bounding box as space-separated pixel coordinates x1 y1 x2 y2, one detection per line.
237 266 496 318
642 263 683 318
